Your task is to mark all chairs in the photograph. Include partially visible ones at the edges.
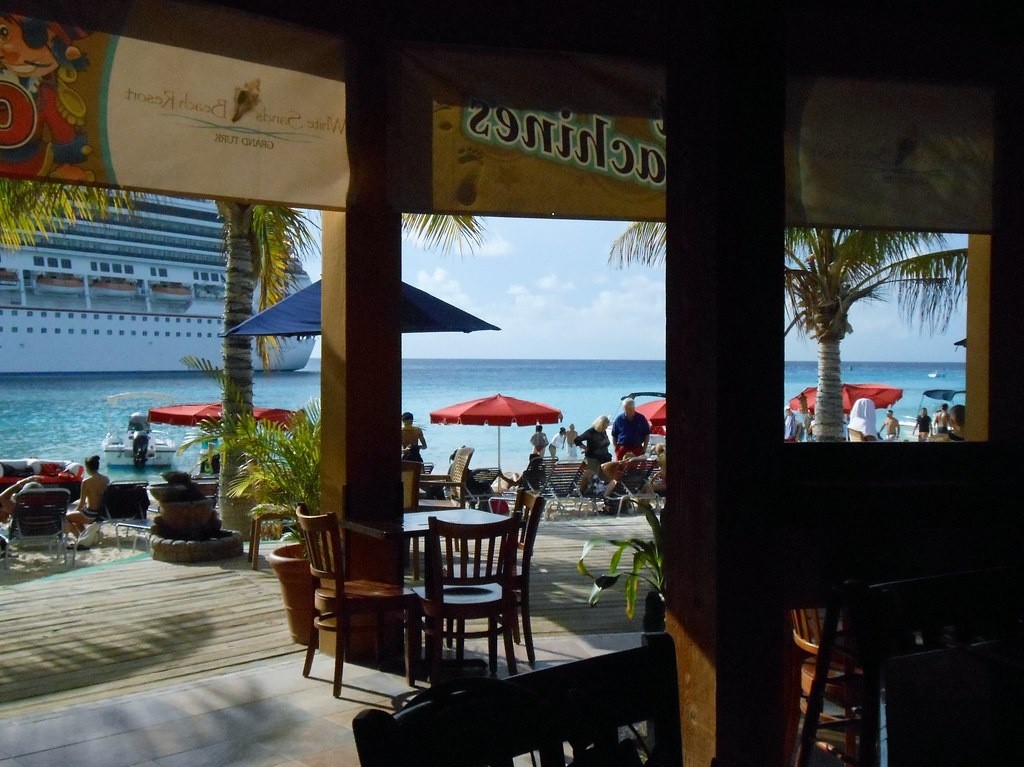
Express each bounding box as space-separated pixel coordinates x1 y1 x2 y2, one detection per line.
847 428 865 442
5 489 70 569
61 480 151 566
252 445 681 767
790 606 868 767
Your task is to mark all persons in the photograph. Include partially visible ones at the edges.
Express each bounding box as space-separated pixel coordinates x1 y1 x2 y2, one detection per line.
912 403 965 441
530 425 549 457
0 475 44 558
63 455 110 547
650 471 665 489
447 447 493 509
651 443 663 455
878 410 900 440
401 412 427 473
562 423 578 458
574 452 637 497
549 427 566 459
133 431 149 468
200 436 220 474
611 397 653 508
497 454 540 489
574 416 611 512
784 406 816 442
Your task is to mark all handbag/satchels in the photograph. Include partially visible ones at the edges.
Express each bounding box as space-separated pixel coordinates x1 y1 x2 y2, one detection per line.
77 522 101 547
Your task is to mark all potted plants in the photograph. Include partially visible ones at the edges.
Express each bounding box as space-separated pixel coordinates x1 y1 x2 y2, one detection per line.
576 497 667 633
173 355 322 648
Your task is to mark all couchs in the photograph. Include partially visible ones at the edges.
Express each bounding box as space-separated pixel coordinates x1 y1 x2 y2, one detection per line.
0 458 84 504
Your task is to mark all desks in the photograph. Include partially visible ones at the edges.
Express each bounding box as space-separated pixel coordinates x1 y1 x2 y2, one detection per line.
339 508 526 664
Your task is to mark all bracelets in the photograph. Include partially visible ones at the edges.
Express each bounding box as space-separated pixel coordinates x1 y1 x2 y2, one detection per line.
619 461 622 466
16 480 23 488
26 477 33 482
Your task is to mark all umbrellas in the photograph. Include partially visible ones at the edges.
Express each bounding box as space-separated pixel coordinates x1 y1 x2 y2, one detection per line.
635 399 666 427
787 383 903 414
219 280 502 336
430 393 563 493
